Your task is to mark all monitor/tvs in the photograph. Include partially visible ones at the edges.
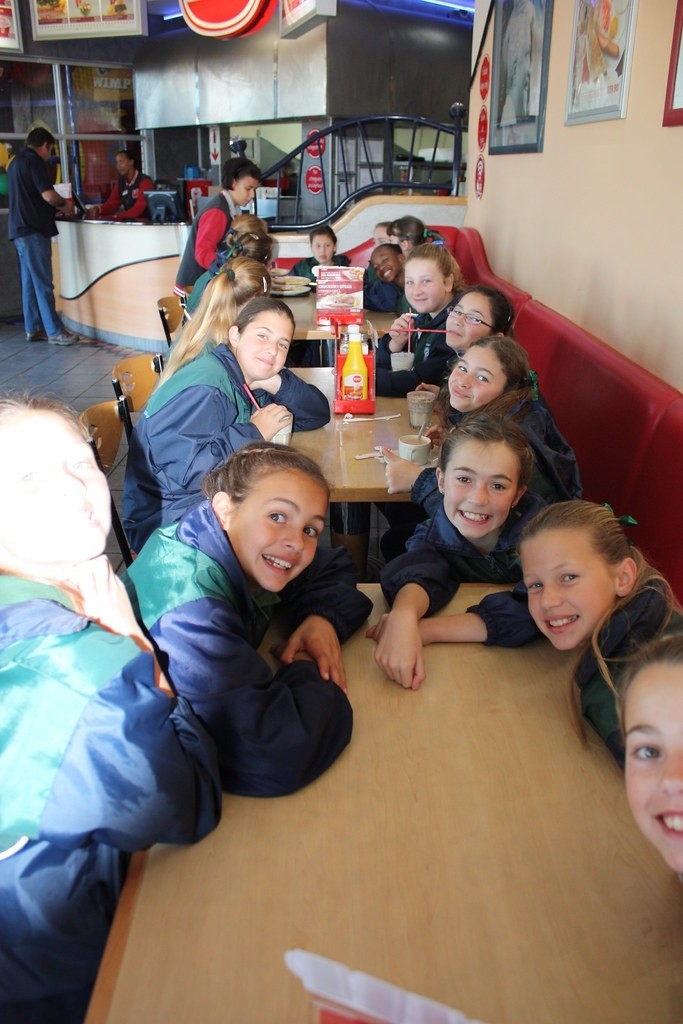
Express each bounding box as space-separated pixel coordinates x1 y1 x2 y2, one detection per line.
142 190 187 224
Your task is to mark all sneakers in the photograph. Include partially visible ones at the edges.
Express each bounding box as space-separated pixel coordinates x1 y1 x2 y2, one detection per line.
27 330 47 340
49 329 79 346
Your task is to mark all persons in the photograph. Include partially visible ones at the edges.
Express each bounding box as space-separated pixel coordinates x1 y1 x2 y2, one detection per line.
9 127 79 344
363 215 551 413
120 441 375 798
0 388 222 1023
288 226 350 293
172 158 263 298
280 161 297 196
384 335 582 516
519 499 683 769
619 637 683 882
89 151 154 221
366 412 544 689
155 214 320 388
121 297 330 555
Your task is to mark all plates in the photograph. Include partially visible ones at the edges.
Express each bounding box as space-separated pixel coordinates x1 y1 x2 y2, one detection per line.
271 275 312 286
269 284 311 296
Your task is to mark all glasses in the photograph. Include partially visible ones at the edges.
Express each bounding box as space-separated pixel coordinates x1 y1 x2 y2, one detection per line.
447 306 494 330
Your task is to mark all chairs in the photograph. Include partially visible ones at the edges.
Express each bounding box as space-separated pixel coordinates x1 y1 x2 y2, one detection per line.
112 353 164 447
78 394 134 569
157 295 189 348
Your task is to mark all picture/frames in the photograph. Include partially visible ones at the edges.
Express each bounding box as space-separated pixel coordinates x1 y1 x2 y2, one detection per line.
488 0 554 155
563 1 639 127
662 0 683 127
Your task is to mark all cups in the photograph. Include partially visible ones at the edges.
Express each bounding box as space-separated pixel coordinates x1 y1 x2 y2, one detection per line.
389 352 415 371
406 390 435 428
255 411 293 449
399 435 432 466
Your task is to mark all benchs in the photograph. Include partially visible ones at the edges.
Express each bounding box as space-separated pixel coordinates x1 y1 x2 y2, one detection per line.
512 298 683 520
623 396 683 615
453 228 532 327
274 225 459 270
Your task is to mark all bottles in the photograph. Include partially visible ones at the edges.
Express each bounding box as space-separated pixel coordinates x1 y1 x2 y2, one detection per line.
340 333 369 400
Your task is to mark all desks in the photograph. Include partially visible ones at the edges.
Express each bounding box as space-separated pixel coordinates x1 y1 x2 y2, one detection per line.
84 582 683 1024
284 367 443 583
269 290 401 368
